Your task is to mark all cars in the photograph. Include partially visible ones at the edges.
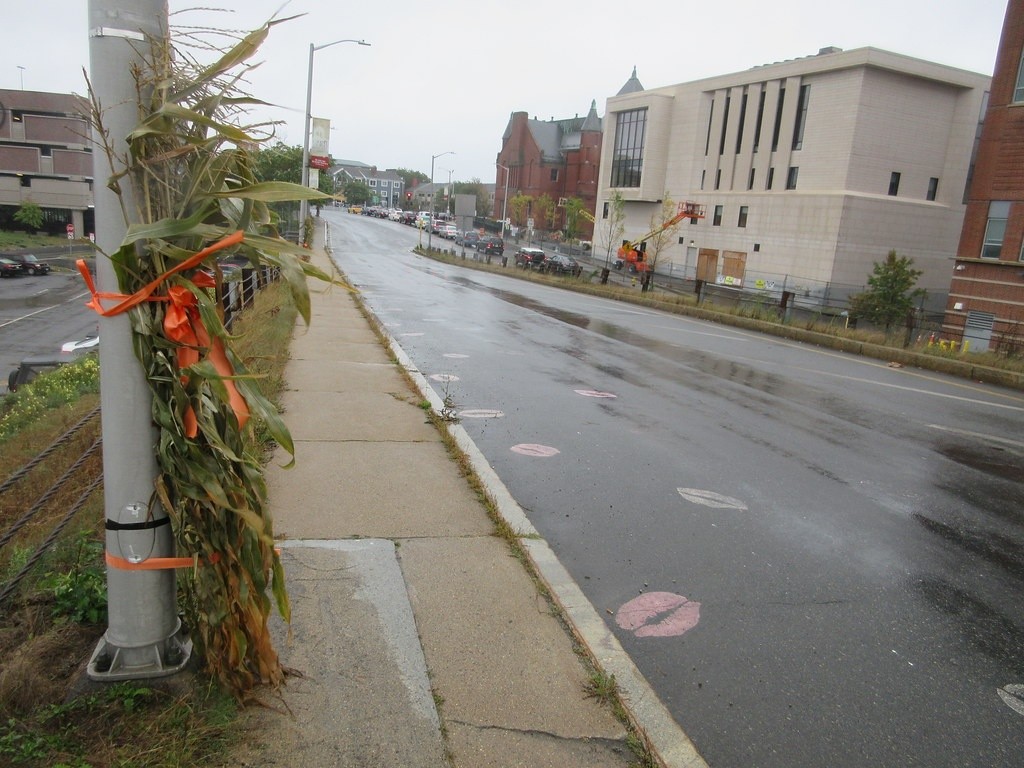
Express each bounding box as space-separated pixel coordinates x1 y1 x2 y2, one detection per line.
514 247 545 271
476 235 504 255
425 219 446 233
579 241 591 250
439 225 457 240
0 252 50 278
539 253 583 278
348 205 434 228
455 231 480 247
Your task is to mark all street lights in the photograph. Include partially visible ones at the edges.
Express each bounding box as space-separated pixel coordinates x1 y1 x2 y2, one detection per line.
297 40 371 247
495 163 510 238
428 151 455 250
